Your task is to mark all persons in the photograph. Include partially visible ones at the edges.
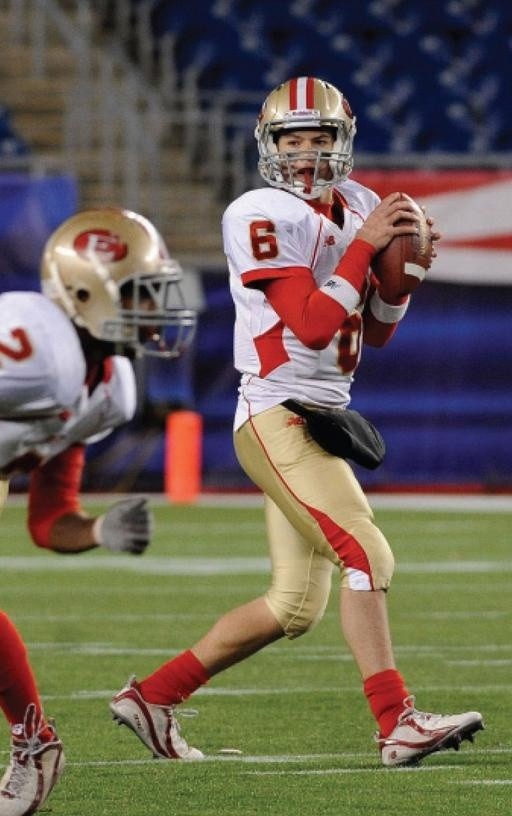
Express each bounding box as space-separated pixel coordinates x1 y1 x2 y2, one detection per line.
0 205 199 816
106 70 489 774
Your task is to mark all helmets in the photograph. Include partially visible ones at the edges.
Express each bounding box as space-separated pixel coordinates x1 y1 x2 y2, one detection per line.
39 208 198 359
253 75 357 200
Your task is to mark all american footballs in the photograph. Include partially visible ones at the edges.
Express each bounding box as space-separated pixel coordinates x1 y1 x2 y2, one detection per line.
370 191 432 297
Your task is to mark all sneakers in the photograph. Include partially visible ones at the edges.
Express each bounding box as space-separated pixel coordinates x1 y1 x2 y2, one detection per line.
0 702 65 814
108 680 205 760
373 695 485 766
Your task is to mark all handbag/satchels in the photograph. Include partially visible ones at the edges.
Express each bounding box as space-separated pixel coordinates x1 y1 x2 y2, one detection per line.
307 408 386 469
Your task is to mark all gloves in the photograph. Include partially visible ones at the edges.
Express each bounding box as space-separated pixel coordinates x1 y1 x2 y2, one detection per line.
102 497 149 553
